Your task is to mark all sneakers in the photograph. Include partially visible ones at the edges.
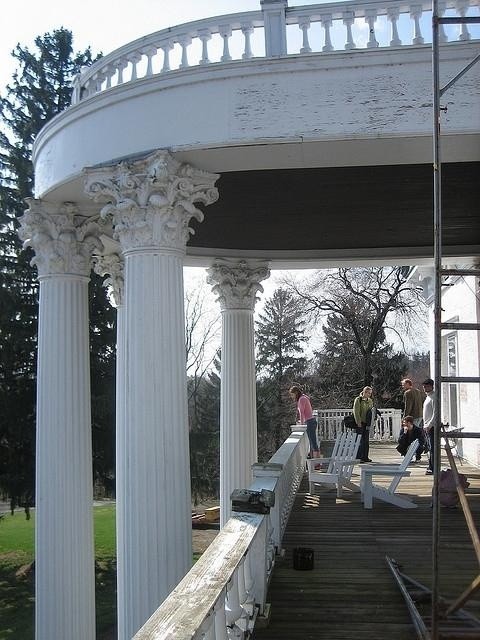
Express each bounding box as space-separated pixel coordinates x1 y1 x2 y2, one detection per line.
410 458 433 475
356 457 373 464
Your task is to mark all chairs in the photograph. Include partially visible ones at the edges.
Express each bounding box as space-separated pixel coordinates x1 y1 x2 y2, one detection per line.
440 426 465 466
359 438 420 509
306 431 362 499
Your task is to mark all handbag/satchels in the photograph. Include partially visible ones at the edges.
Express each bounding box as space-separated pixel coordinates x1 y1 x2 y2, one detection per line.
342 413 360 429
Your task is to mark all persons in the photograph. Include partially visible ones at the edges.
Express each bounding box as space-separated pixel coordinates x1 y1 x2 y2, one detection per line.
288 386 323 470
421 379 439 475
395 414 426 463
399 378 423 463
353 386 377 463
